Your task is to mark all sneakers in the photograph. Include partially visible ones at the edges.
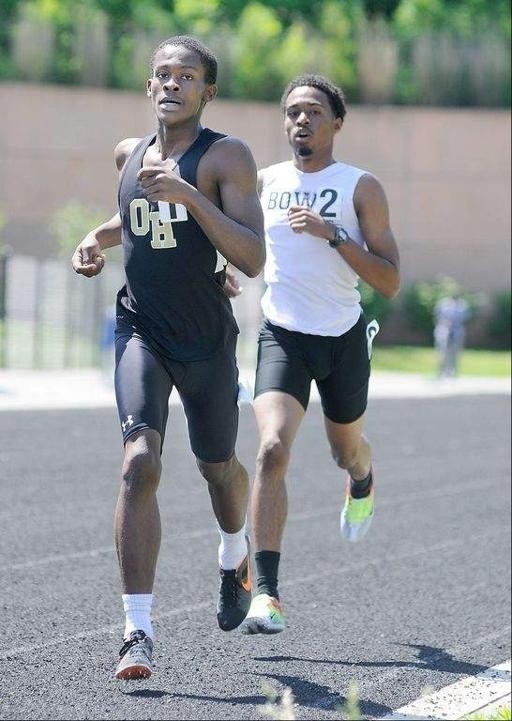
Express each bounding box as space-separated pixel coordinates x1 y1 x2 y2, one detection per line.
340 476 375 542
238 594 284 635
114 630 154 680
216 534 252 631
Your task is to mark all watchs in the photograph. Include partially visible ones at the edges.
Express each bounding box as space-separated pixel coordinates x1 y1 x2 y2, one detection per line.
328 228 349 248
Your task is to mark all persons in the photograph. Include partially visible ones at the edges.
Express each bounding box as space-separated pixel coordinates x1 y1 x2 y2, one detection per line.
433 289 472 381
71 36 266 681
223 75 400 636
